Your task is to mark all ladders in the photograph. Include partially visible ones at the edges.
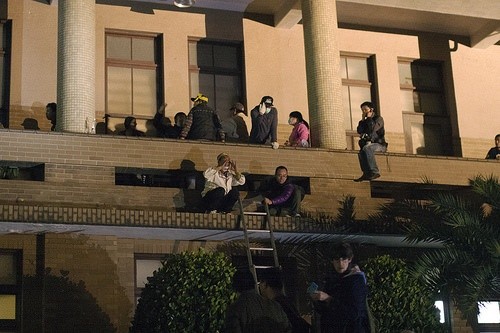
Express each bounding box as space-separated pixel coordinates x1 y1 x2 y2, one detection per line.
238 196 286 297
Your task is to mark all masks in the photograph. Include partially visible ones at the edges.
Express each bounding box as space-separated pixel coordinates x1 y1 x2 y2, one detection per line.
265 108 270 114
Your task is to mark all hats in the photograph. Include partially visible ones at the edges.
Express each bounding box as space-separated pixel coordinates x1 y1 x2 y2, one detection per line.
191 94 208 102
217 152 227 160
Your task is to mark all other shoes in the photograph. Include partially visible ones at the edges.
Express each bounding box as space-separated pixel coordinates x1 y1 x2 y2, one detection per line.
367 173 379 180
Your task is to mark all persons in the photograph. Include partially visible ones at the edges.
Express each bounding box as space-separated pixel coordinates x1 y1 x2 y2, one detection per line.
353 102 389 182
485 135 500 159
258 268 312 333
118 94 251 189
201 152 246 215
219 268 292 333
251 96 280 149
311 243 370 333
264 166 302 218
46 103 56 131
284 112 310 148
